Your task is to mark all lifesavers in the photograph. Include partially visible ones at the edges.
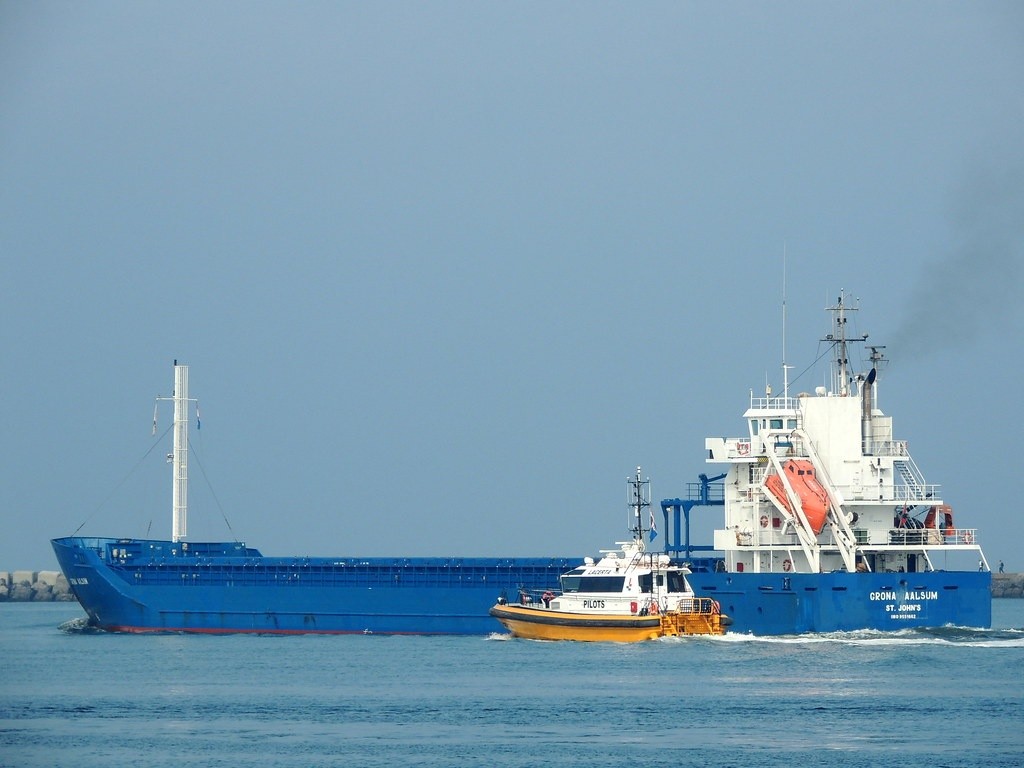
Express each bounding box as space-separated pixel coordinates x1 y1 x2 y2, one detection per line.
543 591 554 602
737 443 748 455
649 603 657 614
759 515 768 527
783 559 792 572
962 533 974 545
713 601 720 615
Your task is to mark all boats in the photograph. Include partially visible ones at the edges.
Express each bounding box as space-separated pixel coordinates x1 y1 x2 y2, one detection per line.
769 463 830 537
49 280 995 640
488 464 735 646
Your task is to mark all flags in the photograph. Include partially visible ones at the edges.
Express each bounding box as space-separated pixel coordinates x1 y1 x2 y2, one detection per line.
901 507 908 524
649 515 657 542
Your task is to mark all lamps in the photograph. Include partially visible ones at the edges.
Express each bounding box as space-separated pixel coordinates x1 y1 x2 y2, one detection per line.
826 335 833 339
863 333 868 338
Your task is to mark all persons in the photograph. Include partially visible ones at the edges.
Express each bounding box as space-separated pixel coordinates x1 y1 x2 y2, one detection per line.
978 560 984 572
997 560 1005 573
543 591 555 602
